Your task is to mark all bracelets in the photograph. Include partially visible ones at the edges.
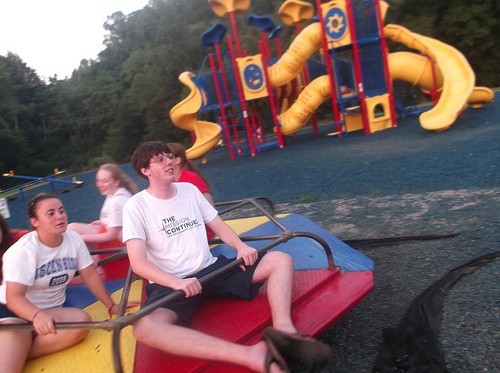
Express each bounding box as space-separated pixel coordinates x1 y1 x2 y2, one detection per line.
108 303 115 319
33 310 42 321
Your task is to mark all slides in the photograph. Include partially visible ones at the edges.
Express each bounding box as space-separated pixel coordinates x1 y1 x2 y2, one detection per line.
266 22 476 131
277 51 494 135
169 72 222 160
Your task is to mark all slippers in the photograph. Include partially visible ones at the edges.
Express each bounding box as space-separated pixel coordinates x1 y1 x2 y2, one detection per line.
262 327 333 373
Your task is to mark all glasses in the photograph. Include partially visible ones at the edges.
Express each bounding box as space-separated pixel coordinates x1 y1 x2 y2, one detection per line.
145 151 177 169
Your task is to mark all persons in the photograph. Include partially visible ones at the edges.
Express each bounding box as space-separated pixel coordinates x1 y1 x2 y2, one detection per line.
166 143 217 242
122 140 332 373
67 163 137 243
0 193 141 373
